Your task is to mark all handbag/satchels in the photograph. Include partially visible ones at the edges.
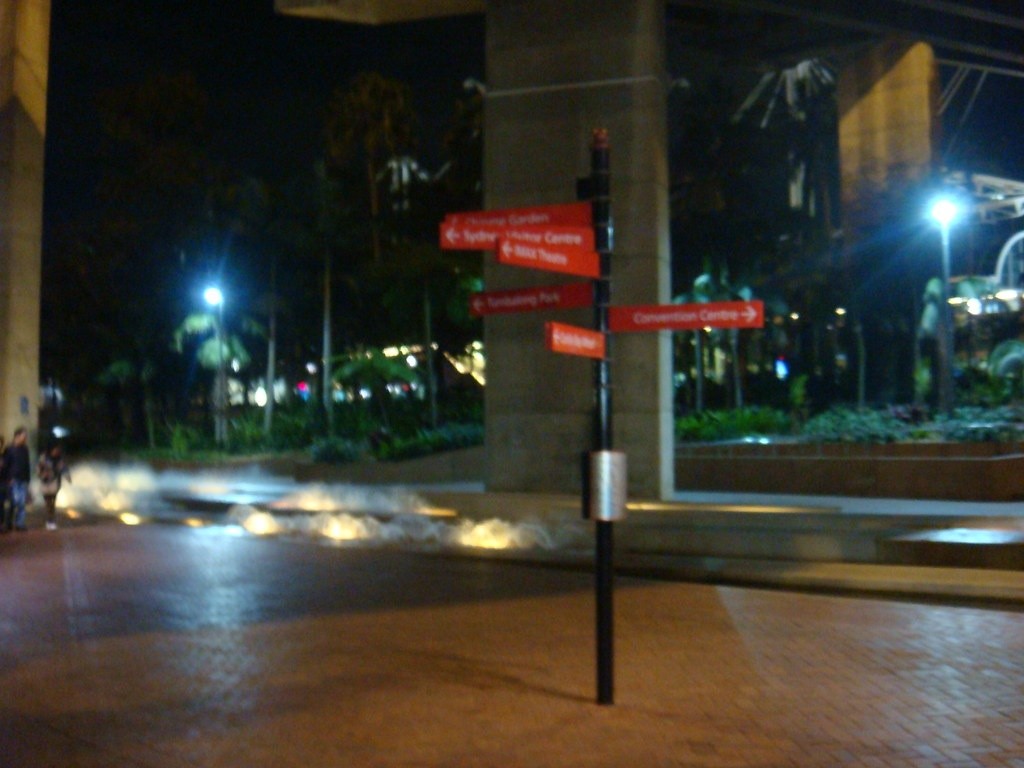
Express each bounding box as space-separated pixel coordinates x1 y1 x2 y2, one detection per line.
40 479 58 496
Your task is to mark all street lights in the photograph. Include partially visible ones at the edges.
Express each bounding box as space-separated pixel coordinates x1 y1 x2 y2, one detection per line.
204 287 224 450
930 198 959 420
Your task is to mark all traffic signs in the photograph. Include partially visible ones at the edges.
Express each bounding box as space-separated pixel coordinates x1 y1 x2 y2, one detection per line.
542 319 606 360
445 205 593 228
438 224 596 254
468 282 596 313
496 233 601 278
607 299 765 334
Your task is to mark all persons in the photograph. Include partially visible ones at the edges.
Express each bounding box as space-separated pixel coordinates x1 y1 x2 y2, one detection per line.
37 441 72 530
0 425 30 530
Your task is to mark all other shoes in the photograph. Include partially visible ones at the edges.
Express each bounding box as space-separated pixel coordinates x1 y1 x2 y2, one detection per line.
6 524 27 531
46 522 57 531
